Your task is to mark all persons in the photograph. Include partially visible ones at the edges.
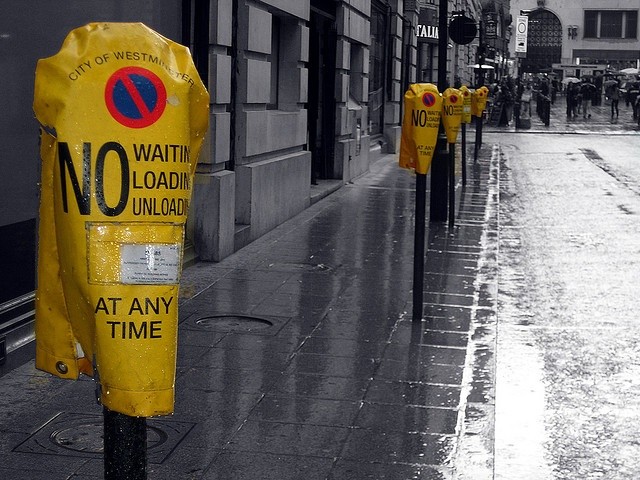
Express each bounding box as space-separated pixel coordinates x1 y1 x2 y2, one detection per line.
594 71 601 106
609 84 619 118
579 83 596 118
566 82 578 118
469 72 558 102
619 75 640 119
581 78 591 82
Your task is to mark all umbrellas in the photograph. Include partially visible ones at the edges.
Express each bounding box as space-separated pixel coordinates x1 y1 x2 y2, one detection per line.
581 75 593 78
561 77 580 85
602 80 618 86
